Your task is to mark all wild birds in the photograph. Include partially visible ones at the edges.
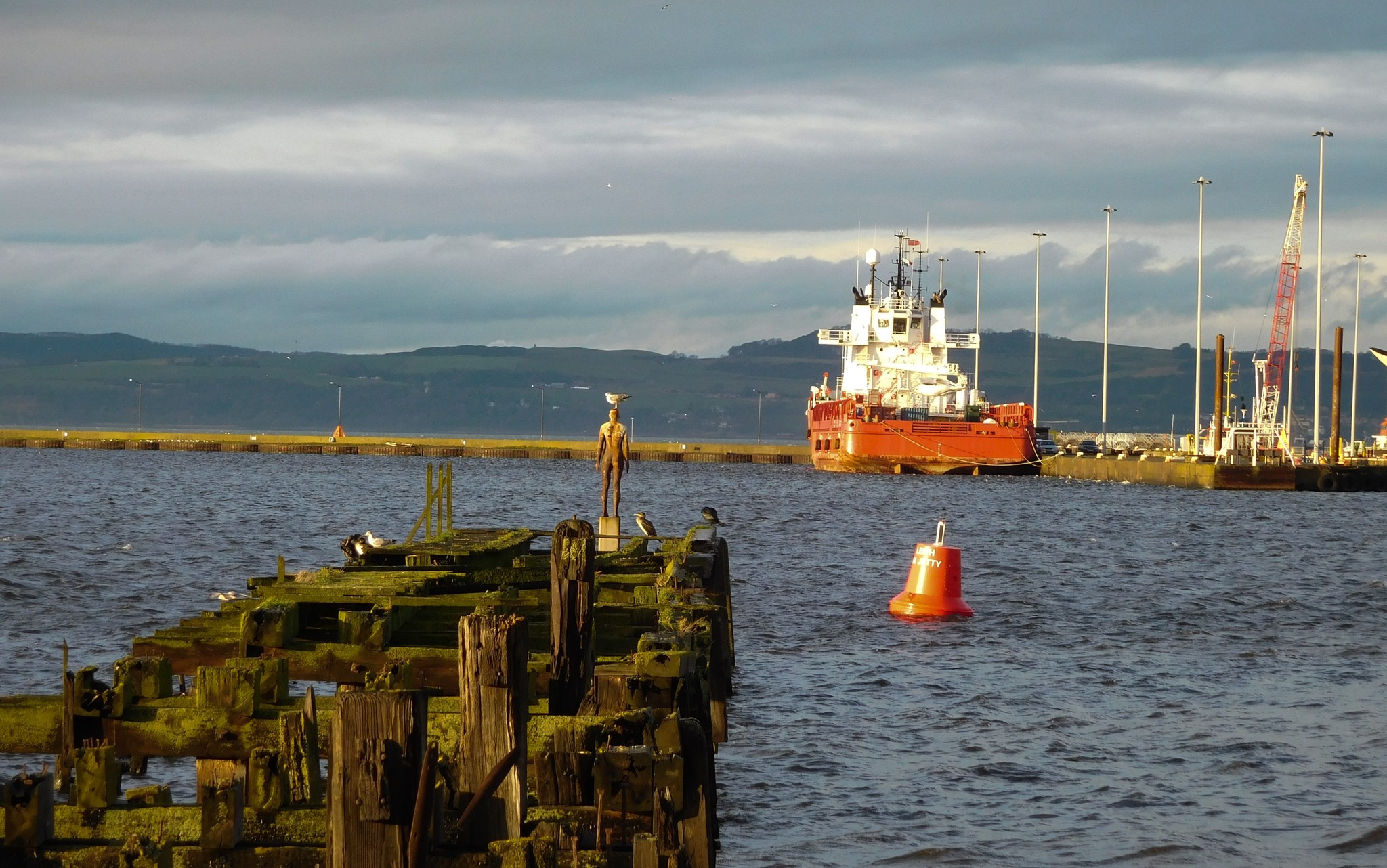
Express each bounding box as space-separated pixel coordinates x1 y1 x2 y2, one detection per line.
633 512 664 544
701 507 729 527
770 304 778 306
341 531 397 563
605 392 632 409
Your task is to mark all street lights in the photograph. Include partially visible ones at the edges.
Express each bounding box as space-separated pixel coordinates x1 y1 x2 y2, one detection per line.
1192 180 1213 454
753 389 761 444
631 417 634 443
1033 233 1048 425
129 379 141 431
330 382 341 437
1100 207 1120 454
973 250 987 388
935 259 949 294
1311 129 1333 463
1349 254 1367 460
532 385 543 440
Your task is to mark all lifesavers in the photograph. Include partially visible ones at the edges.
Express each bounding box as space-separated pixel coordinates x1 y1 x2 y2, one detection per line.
805 429 811 438
816 440 821 450
809 397 813 410
1317 473 1339 492
824 439 829 450
945 405 952 413
835 438 840 449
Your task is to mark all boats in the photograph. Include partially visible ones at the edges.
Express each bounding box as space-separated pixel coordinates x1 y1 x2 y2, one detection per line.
805 228 1037 476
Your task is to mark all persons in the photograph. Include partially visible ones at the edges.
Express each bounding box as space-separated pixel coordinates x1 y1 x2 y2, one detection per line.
596 408 630 517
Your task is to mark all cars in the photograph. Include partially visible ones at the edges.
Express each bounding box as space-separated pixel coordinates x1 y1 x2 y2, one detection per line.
1078 440 1099 455
1037 439 1057 454
